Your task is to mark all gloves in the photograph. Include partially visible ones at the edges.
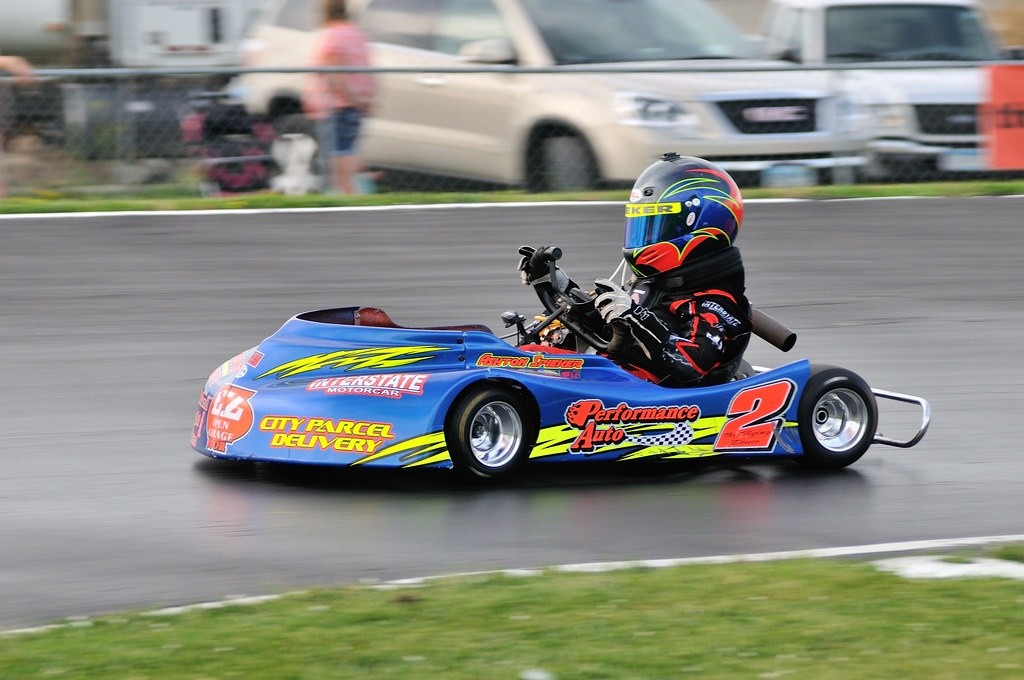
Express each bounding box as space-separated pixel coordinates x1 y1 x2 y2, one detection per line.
593 278 672 361
519 245 582 298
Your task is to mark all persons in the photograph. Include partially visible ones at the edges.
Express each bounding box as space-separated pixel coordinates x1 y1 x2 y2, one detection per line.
518 152 752 389
307 0 384 194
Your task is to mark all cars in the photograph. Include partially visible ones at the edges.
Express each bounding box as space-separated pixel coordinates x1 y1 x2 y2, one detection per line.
761 1 1024 184
219 1 848 190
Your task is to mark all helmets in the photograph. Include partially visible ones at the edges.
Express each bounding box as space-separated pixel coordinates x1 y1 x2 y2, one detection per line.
622 151 744 277
